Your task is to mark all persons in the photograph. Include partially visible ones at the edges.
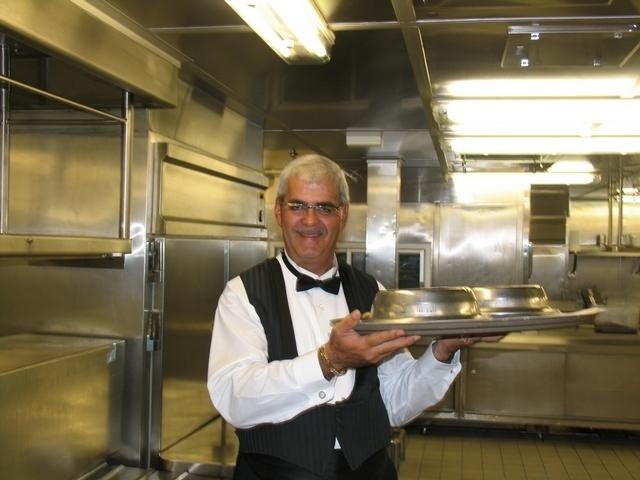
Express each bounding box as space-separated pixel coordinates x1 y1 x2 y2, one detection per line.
205 153 509 478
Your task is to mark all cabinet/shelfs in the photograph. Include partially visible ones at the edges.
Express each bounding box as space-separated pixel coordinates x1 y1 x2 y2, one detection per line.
407 341 460 423
459 343 640 438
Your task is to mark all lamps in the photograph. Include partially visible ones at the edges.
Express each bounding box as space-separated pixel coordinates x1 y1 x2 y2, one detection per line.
224 0 340 68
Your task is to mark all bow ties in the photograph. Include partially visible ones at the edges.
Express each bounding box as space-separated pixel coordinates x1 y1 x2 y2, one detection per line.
279 247 340 294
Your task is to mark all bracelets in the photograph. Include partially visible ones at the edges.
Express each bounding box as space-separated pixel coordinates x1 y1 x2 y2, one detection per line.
318 345 347 377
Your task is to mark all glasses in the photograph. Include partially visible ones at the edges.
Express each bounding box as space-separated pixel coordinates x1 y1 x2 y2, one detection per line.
279 200 342 217
